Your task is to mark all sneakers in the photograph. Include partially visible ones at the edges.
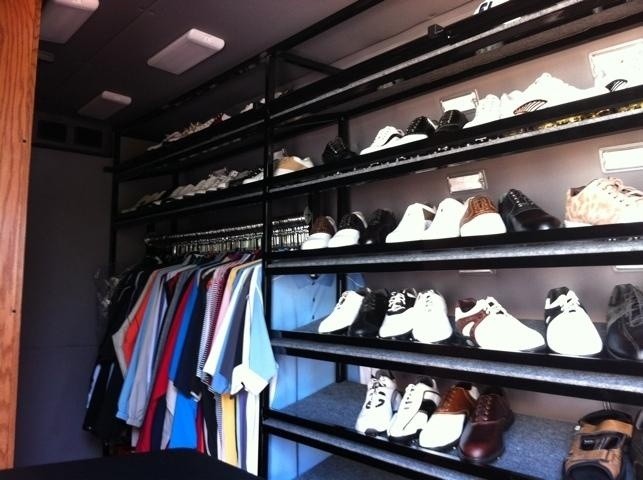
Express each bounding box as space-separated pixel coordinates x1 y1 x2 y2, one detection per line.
459 195 508 239
560 176 642 225
117 148 315 217
383 202 436 245
495 73 610 121
385 375 445 443
298 214 338 250
462 94 514 130
541 287 604 358
315 288 370 334
409 288 453 347
356 207 400 247
450 294 547 353
143 89 287 153
357 126 405 154
378 284 421 341
419 196 468 244
319 137 361 166
353 370 404 439
326 210 369 247
347 290 391 342
393 116 437 148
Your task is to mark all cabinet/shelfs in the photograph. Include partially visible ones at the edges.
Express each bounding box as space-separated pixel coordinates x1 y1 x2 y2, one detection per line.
99 0 643 480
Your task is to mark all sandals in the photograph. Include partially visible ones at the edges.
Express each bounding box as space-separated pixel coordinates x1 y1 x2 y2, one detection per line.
557 406 643 480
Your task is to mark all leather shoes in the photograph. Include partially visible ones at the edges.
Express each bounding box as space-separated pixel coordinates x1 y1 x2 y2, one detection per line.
601 278 643 366
496 188 564 232
456 384 515 466
430 108 470 139
416 379 483 447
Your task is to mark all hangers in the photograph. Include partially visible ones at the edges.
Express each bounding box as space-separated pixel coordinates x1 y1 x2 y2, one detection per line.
106 216 314 286
